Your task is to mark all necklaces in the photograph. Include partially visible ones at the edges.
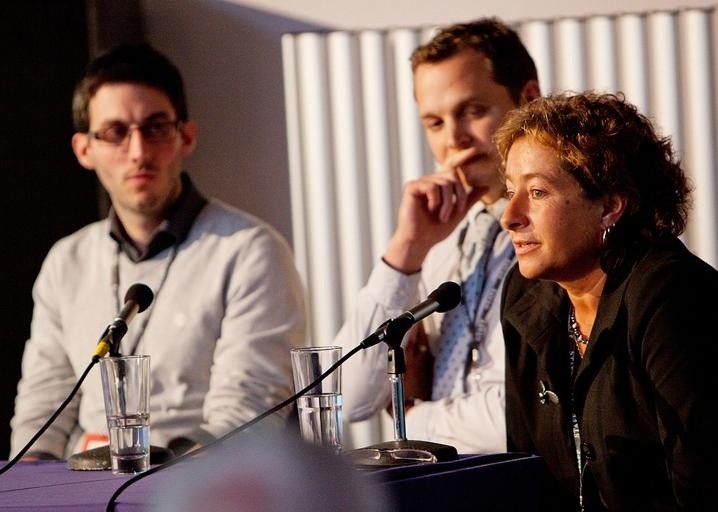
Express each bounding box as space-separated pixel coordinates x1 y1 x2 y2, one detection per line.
566 302 591 349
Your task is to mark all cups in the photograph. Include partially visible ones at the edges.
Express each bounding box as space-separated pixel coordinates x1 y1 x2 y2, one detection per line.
99 355 152 477
289 346 342 451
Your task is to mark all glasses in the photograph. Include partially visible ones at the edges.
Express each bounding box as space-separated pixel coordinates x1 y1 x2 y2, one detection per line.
82 115 185 146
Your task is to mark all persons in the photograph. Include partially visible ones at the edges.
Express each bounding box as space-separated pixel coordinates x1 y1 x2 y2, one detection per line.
317 13 547 459
8 44 311 471
492 90 718 512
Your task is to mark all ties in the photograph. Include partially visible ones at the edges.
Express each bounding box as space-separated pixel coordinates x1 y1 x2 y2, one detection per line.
427 207 502 401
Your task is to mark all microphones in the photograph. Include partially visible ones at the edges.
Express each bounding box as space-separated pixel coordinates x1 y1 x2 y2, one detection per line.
91 283 155 363
358 282 461 349
